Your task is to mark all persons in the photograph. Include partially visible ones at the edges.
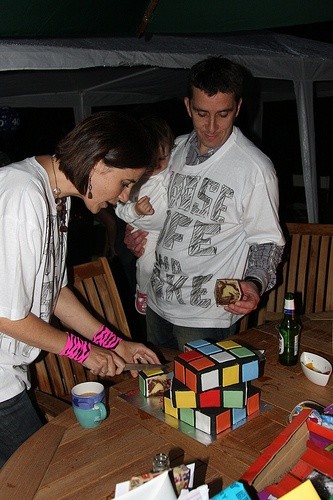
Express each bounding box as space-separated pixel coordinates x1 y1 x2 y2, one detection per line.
114 57 287 356
0 111 158 464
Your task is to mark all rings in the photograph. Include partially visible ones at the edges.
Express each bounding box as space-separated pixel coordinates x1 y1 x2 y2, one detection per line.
130 245 134 250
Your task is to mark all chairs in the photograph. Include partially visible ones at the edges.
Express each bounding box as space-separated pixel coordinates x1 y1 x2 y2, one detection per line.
29 256 132 421
240 223 333 333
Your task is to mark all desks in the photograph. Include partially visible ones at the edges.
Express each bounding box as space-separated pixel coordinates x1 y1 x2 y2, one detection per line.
0 311 333 500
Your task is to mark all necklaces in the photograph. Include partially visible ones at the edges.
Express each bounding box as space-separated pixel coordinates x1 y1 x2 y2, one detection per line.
50 156 69 231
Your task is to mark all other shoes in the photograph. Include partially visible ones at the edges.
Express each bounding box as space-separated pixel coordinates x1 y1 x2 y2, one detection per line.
134 290 148 316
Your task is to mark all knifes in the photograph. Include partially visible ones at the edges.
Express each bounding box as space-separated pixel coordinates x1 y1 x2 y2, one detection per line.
122 363 169 372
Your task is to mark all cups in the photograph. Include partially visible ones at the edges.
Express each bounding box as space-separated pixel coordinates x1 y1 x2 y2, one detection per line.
71 381 107 428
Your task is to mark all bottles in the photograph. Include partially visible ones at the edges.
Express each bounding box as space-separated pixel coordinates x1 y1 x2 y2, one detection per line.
151 453 170 471
278 293 299 366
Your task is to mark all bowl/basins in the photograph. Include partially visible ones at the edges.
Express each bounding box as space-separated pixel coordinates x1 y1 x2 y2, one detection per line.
301 351 332 387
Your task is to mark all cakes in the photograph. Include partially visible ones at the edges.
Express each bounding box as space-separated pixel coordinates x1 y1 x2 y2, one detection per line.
214 279 242 307
139 338 261 436
